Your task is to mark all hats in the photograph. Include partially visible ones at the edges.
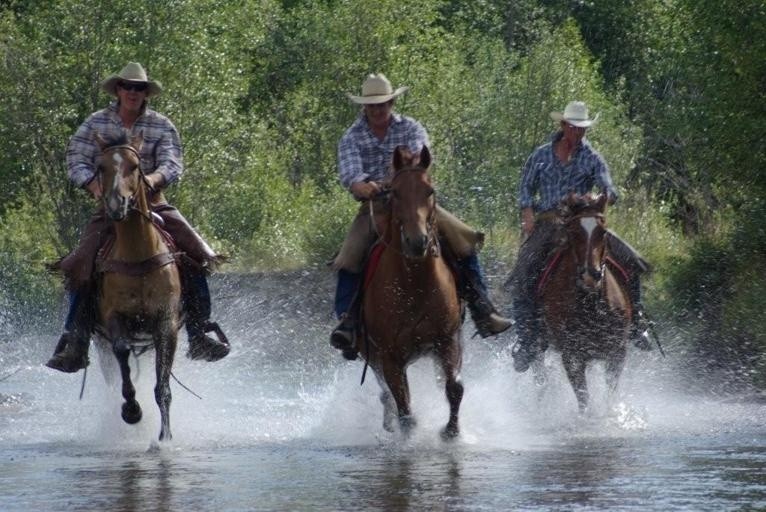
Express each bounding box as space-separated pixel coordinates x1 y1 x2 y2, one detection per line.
101 62 161 96
549 101 599 127
350 72 408 104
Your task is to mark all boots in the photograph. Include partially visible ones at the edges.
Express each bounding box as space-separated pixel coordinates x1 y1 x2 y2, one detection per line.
463 283 516 338
188 327 229 360
47 332 88 369
331 308 360 360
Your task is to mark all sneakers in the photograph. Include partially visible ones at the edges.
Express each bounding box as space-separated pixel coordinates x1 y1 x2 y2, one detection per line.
513 338 548 372
630 330 654 351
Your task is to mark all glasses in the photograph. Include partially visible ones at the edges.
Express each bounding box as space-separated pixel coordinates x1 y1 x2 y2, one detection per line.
122 83 144 91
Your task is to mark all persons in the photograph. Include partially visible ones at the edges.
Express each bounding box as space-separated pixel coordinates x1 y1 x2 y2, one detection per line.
330 78 513 363
46 65 230 372
511 105 657 374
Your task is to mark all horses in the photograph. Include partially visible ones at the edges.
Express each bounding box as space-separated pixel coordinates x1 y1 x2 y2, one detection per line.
42 128 187 442
531 185 633 413
354 144 467 441
